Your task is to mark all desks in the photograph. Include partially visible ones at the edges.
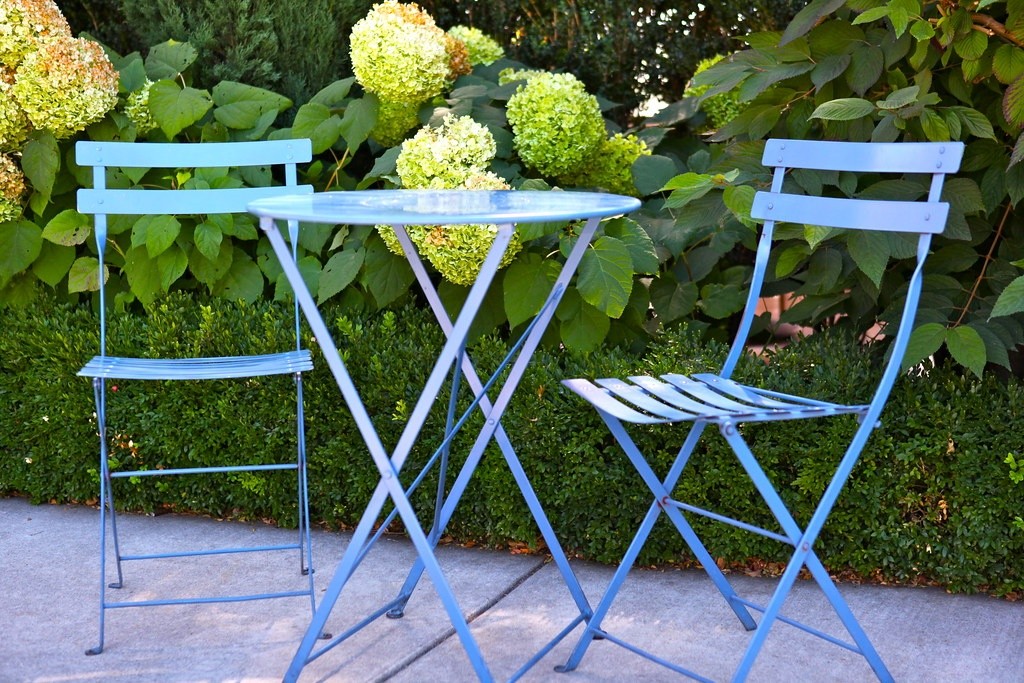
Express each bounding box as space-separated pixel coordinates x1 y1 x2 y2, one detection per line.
247 190 641 683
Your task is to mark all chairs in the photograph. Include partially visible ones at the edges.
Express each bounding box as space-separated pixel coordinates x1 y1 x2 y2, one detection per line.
555 139 964 683
76 138 332 655
